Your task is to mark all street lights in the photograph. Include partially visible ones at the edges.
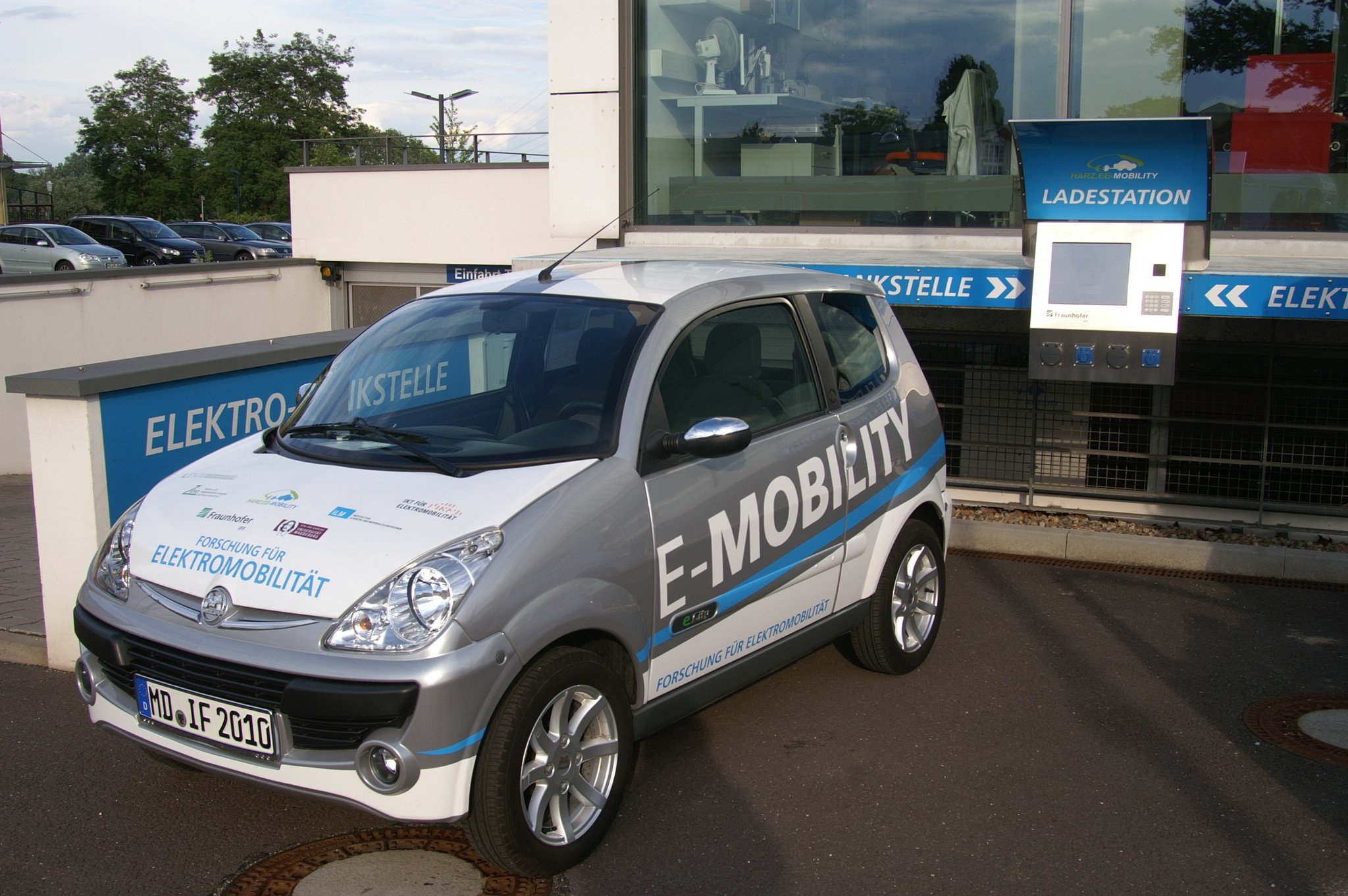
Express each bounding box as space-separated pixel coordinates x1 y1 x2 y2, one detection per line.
46 180 54 222
403 89 479 164
230 169 240 216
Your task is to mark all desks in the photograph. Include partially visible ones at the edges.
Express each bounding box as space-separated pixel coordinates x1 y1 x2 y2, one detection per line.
660 93 915 226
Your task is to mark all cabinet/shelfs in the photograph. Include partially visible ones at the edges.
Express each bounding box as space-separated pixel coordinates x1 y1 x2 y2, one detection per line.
1229 52 1348 232
740 142 837 176
658 0 845 48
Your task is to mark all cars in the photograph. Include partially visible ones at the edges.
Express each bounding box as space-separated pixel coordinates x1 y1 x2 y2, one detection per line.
241 221 292 247
69 258 954 882
0 222 127 279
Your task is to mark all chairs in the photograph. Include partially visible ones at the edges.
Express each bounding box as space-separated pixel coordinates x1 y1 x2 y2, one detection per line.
45 232 56 240
27 231 37 244
667 322 779 427
883 69 999 228
531 328 671 434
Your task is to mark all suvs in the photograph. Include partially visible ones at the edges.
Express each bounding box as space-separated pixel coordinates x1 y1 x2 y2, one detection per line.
164 220 293 263
63 215 207 267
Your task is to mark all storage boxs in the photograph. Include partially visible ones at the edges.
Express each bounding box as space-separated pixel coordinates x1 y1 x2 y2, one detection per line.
797 211 864 225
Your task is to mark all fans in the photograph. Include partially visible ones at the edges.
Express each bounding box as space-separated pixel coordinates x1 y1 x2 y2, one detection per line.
695 16 741 95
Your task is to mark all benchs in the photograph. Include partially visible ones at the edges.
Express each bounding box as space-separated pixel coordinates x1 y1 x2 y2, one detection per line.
4 230 19 244
628 322 858 420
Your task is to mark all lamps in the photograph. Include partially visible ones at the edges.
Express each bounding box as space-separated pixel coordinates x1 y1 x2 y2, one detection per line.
320 262 342 282
